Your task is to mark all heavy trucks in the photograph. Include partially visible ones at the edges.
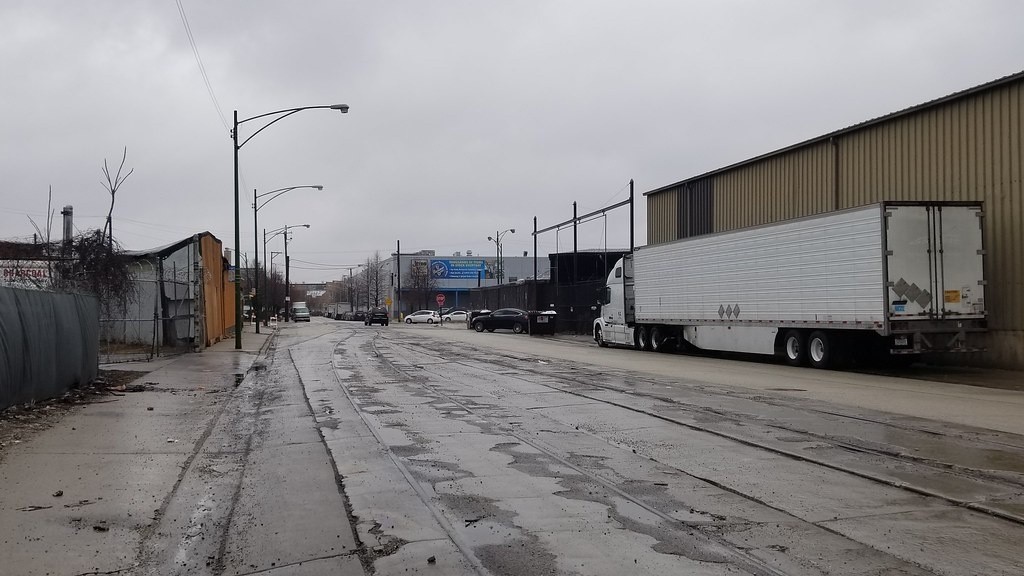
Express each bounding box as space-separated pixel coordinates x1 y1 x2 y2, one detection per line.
593 199 991 372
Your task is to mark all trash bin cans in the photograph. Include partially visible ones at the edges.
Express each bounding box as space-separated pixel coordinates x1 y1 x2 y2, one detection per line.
527 310 558 336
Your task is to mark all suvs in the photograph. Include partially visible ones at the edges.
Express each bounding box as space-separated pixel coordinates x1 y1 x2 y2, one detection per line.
364 307 389 326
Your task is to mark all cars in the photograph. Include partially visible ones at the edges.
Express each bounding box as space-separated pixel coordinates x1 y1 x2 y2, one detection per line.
276 301 367 322
472 308 529 334
403 308 441 323
441 310 470 323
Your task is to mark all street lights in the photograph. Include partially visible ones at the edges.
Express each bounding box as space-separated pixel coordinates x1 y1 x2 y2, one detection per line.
488 229 516 284
232 103 350 349
261 223 314 328
254 184 323 336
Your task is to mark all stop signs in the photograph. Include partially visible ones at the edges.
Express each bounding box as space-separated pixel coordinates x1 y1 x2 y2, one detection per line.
435 294 445 305
